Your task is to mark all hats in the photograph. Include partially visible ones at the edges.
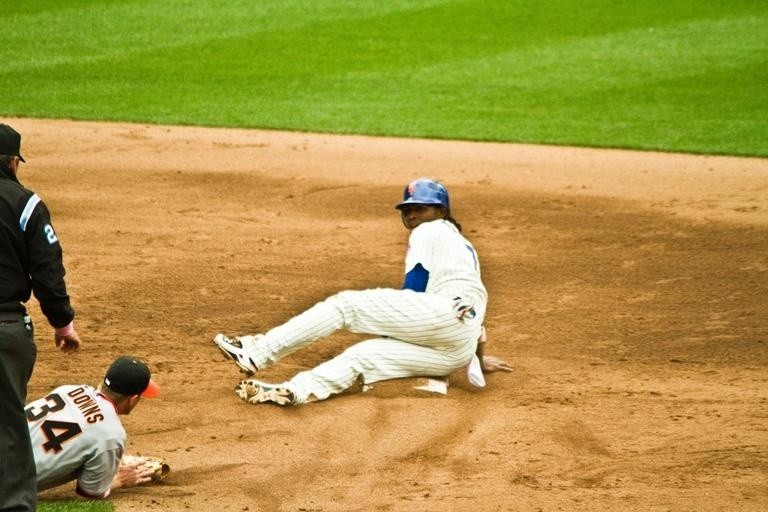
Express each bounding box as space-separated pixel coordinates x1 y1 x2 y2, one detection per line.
0 122 26 164
105 356 160 397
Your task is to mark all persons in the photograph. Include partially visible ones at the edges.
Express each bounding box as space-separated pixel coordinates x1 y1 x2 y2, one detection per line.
212 179 514 407
0 123 81 512
23 355 155 500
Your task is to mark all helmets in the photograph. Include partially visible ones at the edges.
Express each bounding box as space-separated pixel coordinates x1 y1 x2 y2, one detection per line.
397 178 451 212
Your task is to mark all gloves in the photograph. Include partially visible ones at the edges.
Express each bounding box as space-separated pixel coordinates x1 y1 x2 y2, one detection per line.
450 296 475 319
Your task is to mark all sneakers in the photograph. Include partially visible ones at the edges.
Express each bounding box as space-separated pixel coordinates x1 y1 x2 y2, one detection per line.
236 379 297 408
214 333 258 376
